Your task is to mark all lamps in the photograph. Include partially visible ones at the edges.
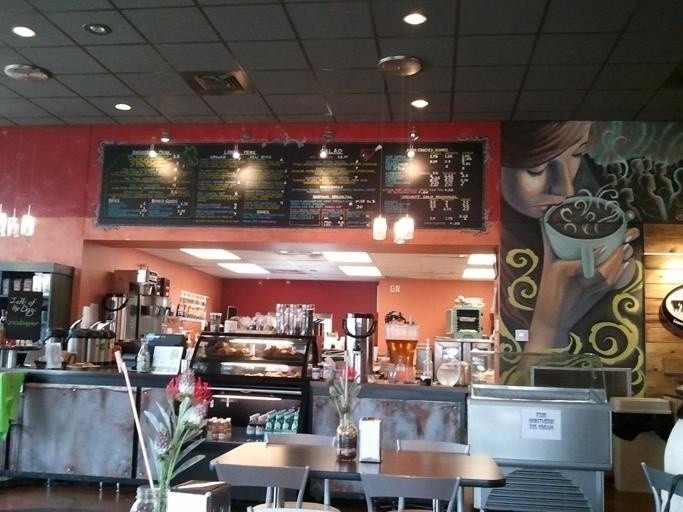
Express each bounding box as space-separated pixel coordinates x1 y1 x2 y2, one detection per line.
373 56 422 245
408 129 420 158
320 129 334 158
0 63 50 237
232 131 251 158
149 134 170 157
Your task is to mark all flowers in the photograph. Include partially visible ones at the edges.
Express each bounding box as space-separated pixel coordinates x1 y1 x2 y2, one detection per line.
144 370 211 512
328 359 362 428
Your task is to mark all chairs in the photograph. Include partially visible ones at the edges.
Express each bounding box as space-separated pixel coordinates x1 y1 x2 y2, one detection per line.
641 462 683 512
213 461 309 512
253 432 340 512
360 472 460 512
388 439 470 512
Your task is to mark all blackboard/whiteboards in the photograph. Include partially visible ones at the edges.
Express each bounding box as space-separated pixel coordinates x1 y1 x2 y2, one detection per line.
95 138 489 232
5 290 43 341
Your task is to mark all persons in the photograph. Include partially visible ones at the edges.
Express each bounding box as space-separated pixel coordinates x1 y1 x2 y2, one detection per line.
502 117 650 396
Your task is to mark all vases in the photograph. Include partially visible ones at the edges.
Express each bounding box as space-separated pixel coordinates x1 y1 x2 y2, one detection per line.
336 418 358 462
130 484 170 512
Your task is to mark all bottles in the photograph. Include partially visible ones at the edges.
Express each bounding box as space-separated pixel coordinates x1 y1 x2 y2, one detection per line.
207 416 231 441
422 346 433 383
246 410 301 442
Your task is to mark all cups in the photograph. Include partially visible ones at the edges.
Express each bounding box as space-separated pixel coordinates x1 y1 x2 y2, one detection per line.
209 312 221 332
542 194 628 283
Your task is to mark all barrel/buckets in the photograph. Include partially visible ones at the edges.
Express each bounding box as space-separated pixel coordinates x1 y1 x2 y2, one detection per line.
100 293 130 342
341 310 378 384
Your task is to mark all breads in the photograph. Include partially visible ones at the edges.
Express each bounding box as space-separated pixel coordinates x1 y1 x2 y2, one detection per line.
221 345 301 378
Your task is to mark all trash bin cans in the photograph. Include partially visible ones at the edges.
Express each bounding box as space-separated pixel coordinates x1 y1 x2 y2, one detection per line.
166 479 231 512
